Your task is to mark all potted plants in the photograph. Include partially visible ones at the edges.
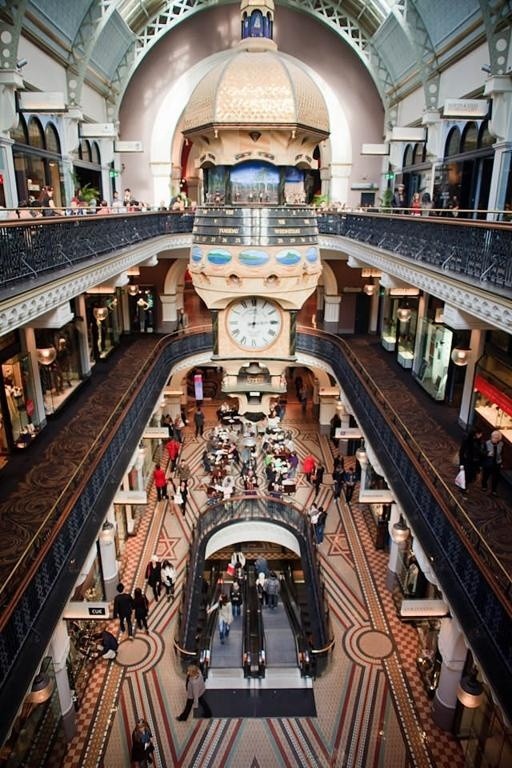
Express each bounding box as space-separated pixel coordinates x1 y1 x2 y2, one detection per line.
77 182 102 209
377 190 394 213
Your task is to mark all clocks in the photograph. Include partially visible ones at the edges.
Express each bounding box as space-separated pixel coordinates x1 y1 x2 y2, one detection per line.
225 297 285 353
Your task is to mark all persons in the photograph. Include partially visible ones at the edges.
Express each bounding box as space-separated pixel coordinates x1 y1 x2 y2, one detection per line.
13 388 26 431
178 308 188 329
206 593 234 644
38 338 73 396
75 555 177 660
8 186 147 220
254 553 281 610
130 719 158 768
153 376 359 546
230 547 246 617
158 196 185 211
459 424 504 497
391 183 460 218
176 664 212 721
136 290 154 333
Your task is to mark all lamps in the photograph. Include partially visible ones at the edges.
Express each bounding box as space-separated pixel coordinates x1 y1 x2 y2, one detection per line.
93 294 108 320
27 673 54 705
363 275 377 297
392 512 409 543
101 518 116 540
125 275 140 297
355 439 368 463
36 329 57 366
456 664 484 708
396 288 412 322
451 329 472 366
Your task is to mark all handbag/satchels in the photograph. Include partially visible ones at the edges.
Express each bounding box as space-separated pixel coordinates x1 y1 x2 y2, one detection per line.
173 487 184 504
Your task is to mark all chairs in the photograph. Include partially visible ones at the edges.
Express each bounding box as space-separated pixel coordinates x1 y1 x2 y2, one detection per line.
201 407 299 500
